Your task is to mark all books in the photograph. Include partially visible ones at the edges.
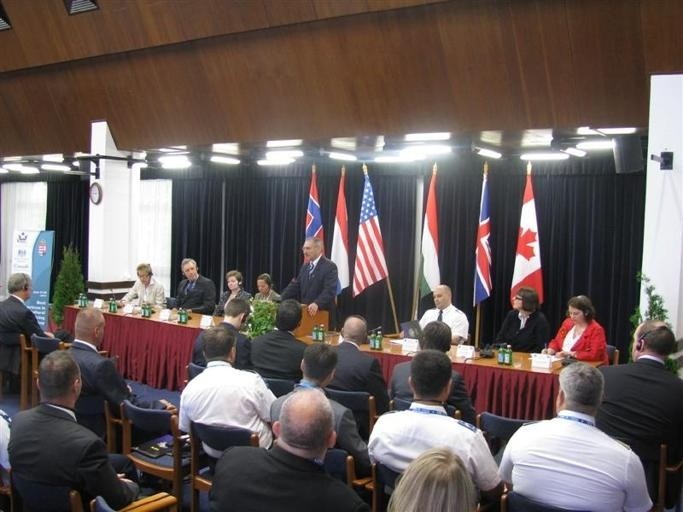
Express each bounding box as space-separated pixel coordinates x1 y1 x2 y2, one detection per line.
136 437 171 459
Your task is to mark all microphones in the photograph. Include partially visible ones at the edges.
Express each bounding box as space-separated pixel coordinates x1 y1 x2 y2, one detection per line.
479 336 502 359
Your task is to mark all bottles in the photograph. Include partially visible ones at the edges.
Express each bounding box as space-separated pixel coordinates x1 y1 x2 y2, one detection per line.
141 303 146 317
78 292 87 308
369 331 376 349
145 303 151 317
375 331 383 350
504 344 513 365
177 307 183 323
497 344 505 364
312 324 319 340
318 324 325 342
108 297 118 313
180 308 188 324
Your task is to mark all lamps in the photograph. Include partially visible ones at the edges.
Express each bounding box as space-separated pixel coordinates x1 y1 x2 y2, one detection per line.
519 152 570 162
471 146 502 160
575 126 638 136
560 145 587 159
323 150 358 162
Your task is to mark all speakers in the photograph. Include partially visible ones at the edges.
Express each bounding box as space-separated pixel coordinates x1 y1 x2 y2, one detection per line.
611 133 646 175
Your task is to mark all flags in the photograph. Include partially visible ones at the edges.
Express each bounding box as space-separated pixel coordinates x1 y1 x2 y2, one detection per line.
419 176 440 288
325 175 351 292
510 172 544 312
472 175 493 308
302 172 325 265
353 174 388 297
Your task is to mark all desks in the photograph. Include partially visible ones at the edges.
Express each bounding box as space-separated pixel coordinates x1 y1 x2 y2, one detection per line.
63 299 605 421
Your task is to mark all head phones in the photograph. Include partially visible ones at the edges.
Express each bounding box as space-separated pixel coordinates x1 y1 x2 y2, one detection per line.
136 263 153 278
635 328 679 353
239 272 245 287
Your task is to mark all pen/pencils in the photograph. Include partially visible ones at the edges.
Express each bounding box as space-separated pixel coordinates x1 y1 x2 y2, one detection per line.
544 343 548 356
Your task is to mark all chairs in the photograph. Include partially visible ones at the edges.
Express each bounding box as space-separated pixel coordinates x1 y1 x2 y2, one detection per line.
613 435 683 512
119 399 208 512
365 461 403 512
388 397 462 421
89 495 179 512
30 333 109 411
475 411 536 468
188 419 259 512
324 387 381 445
323 448 372 490
184 362 206 387
605 344 620 366
263 377 296 398
0 332 73 410
7 467 169 512
75 396 121 454
499 490 569 512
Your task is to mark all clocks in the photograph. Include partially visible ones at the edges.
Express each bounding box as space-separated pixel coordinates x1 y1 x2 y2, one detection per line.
89 181 103 205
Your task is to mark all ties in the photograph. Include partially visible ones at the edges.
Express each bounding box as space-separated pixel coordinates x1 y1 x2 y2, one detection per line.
437 309 443 322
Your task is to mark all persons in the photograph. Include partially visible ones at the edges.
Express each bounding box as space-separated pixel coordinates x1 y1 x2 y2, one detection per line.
118 264 165 308
367 350 506 504
597 320 682 469
497 289 552 354
272 238 338 317
70 309 174 428
270 344 369 478
210 389 369 512
387 448 477 511
497 364 657 512
174 259 216 314
8 350 140 512
254 274 276 303
214 272 251 317
418 283 469 345
0 272 73 360
542 295 609 363
179 321 279 458
